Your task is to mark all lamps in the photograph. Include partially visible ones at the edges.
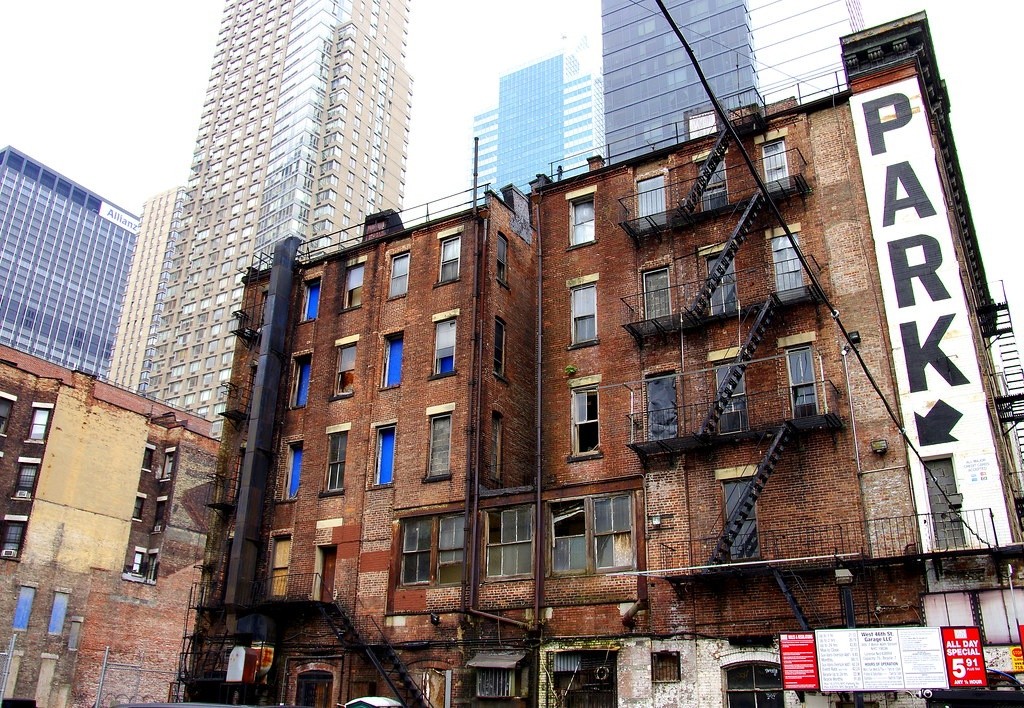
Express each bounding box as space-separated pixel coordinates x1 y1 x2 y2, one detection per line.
871 439 888 455
835 568 854 586
847 331 861 346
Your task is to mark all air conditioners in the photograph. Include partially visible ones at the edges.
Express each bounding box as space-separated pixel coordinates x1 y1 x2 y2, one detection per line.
154 525 161 531
16 490 27 499
3 550 14 557
